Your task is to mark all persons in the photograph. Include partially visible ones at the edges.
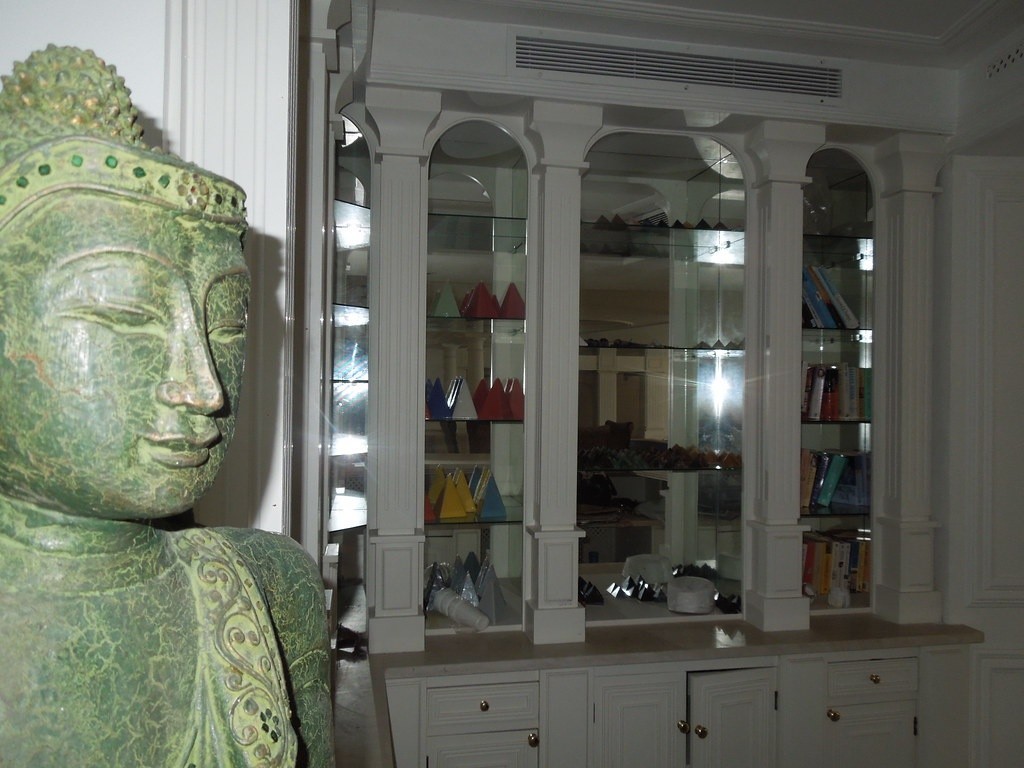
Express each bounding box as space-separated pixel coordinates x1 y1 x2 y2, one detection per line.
0 40 337 768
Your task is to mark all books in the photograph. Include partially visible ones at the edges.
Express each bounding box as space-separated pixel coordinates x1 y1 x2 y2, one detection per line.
801 263 858 329
801 361 872 422
802 531 871 595
799 447 870 507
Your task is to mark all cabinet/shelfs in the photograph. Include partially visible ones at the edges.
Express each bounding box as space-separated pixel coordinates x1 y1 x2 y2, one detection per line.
337 79 985 768
